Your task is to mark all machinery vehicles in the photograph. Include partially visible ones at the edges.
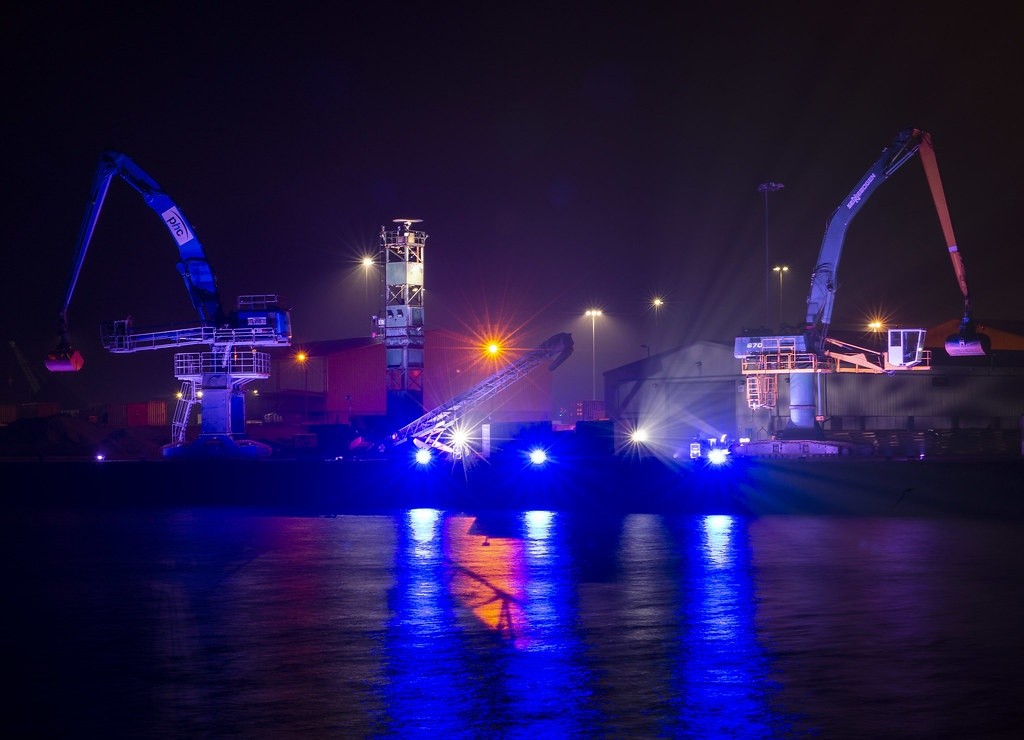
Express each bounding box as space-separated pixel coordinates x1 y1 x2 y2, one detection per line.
729 127 991 371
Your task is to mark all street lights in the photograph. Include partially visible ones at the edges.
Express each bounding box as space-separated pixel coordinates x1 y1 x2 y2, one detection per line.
653 297 662 358
639 345 649 357
297 351 309 392
772 266 790 330
585 309 602 400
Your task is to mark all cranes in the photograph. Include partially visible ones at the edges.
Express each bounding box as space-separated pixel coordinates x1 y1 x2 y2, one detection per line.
38 151 294 462
374 329 579 468
